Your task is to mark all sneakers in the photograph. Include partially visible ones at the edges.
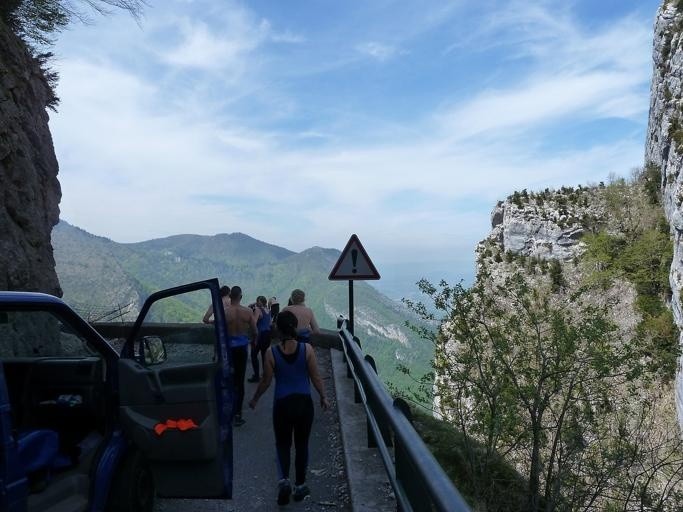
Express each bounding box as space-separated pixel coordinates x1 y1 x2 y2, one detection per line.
294 486 312 503
234 419 245 426
277 480 292 504
247 375 259 383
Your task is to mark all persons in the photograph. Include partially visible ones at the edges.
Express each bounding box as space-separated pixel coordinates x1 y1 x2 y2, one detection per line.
246 309 328 507
203 283 318 426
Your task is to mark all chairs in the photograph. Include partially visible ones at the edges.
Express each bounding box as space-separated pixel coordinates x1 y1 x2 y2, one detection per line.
0 360 61 480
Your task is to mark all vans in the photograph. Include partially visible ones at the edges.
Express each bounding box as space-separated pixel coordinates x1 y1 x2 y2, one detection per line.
0 277 238 511
